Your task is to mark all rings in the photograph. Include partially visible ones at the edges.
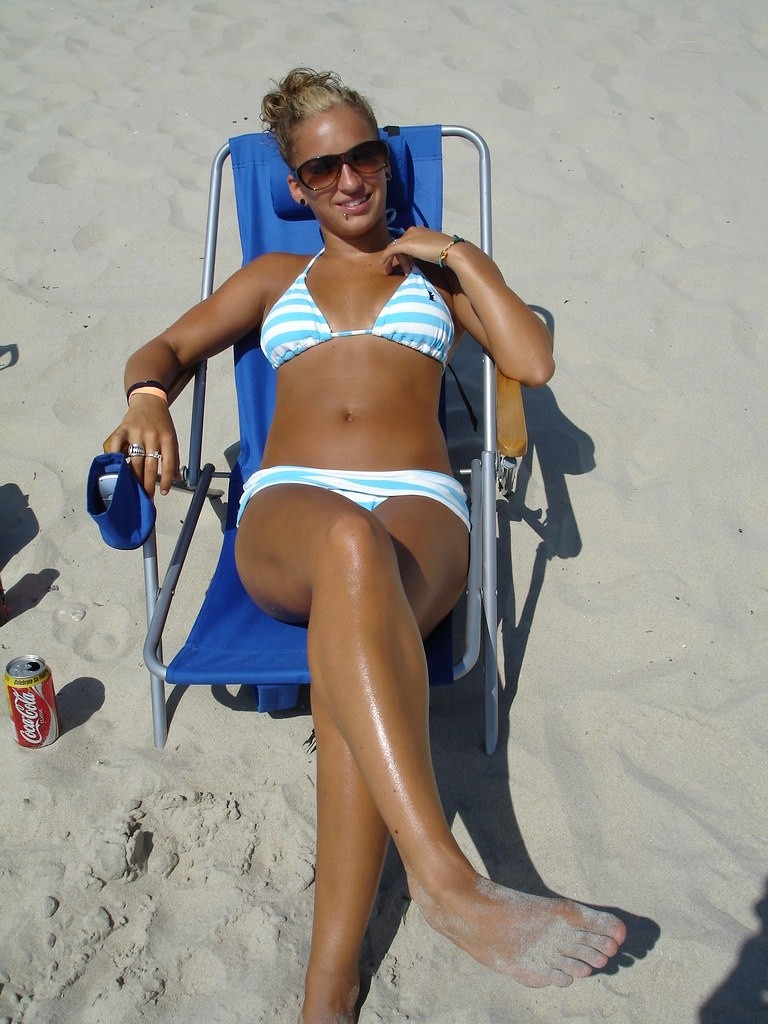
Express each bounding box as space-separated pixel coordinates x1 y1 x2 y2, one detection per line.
146 451 161 459
393 239 397 246
128 444 145 457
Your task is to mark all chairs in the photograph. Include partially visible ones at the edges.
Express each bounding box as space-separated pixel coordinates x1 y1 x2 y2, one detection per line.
86 124 528 754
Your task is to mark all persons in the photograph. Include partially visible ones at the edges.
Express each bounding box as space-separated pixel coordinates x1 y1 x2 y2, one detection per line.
104 68 631 1024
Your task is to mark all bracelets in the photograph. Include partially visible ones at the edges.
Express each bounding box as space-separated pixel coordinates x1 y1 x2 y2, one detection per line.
440 234 466 268
127 381 167 406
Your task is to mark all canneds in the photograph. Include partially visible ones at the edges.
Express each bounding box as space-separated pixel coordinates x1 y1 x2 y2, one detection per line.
3 655 60 748
